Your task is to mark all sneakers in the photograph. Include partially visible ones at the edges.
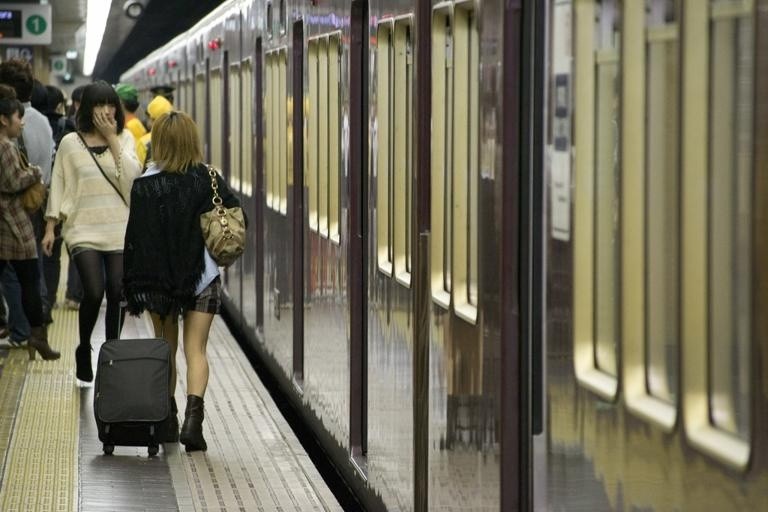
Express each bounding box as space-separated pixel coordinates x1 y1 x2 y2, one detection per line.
0 334 28 347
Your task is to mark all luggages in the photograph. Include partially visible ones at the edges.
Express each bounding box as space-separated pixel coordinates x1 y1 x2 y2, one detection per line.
93 300 172 457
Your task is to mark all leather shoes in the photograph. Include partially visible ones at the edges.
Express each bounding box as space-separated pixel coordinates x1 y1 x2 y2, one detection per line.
76 344 93 382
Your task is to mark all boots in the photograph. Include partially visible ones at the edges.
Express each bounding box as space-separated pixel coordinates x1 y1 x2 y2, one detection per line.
157 397 179 444
181 394 208 453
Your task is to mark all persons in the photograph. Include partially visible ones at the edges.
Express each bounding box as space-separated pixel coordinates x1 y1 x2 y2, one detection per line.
118 85 173 172
43 81 141 381
1 60 84 361
122 112 248 455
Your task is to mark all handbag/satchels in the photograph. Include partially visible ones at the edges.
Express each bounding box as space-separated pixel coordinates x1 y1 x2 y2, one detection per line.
20 174 48 215
201 206 247 267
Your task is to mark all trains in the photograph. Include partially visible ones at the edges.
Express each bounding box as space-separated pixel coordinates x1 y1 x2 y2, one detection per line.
119 0 768 512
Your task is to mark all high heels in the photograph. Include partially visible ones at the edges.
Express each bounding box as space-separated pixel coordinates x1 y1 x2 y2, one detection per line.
28 323 61 360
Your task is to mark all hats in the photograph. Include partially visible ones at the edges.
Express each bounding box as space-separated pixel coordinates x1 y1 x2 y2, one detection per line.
147 95 173 119
116 84 141 102
